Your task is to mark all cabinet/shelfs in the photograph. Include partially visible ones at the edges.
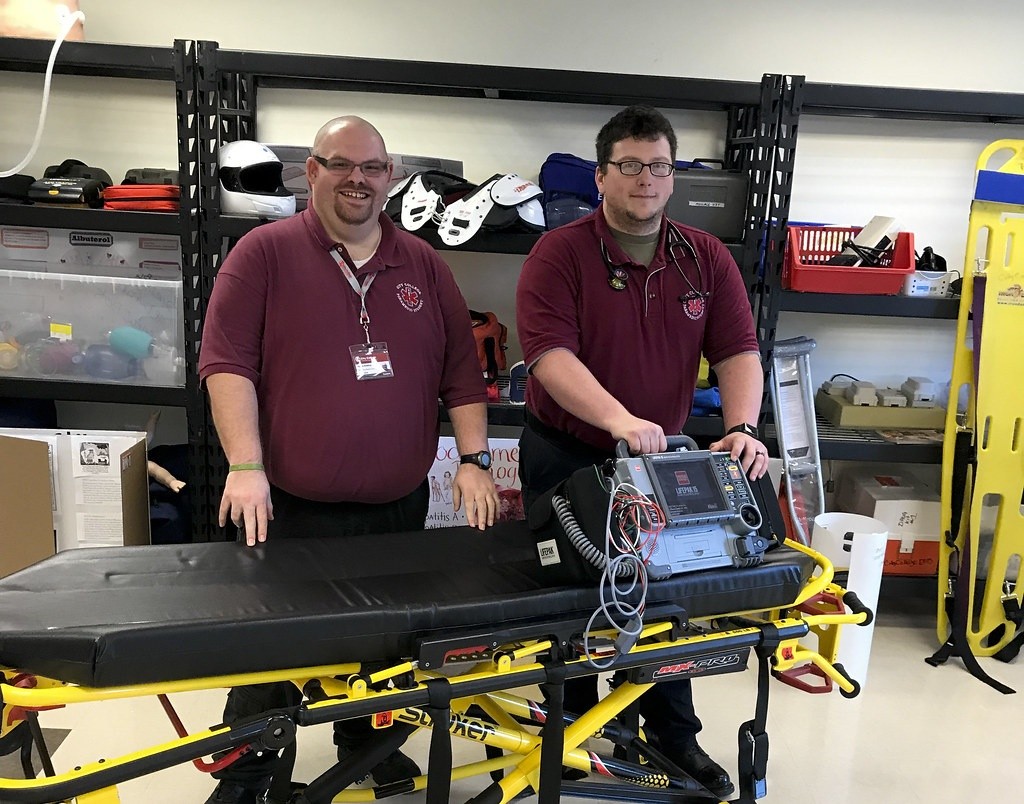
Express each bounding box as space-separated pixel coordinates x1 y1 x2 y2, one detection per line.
0 34 1024 598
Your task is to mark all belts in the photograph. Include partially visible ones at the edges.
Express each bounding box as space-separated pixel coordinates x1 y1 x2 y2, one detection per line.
526 411 615 465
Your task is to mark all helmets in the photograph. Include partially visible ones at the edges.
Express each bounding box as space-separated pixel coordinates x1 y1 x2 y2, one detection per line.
218 140 296 219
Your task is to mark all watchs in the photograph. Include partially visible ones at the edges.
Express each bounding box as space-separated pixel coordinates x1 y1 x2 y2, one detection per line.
727 422 760 440
460 450 493 471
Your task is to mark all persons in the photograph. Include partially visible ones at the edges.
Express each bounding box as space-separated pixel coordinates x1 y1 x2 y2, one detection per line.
198 115 502 804
519 106 766 798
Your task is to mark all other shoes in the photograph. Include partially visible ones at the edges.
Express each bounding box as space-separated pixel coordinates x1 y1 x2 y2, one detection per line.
337 744 423 794
204 778 264 804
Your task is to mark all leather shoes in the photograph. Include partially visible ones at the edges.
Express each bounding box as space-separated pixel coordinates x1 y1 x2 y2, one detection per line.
660 736 735 797
512 726 589 797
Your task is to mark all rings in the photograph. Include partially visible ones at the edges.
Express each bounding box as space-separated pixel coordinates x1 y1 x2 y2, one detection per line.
756 451 764 456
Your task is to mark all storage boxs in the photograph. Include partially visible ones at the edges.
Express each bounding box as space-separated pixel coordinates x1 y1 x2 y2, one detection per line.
0 269 216 387
905 271 951 298
756 219 836 273
816 387 947 430
976 494 1024 580
664 158 749 240
836 467 942 575
782 225 916 295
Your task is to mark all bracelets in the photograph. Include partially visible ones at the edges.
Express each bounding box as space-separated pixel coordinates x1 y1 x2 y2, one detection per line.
229 462 264 471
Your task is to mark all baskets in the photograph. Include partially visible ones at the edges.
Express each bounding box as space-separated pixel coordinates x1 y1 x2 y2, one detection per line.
772 227 913 298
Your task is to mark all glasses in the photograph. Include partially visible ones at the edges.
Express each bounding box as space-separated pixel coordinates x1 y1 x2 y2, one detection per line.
313 154 389 178
604 159 675 177
